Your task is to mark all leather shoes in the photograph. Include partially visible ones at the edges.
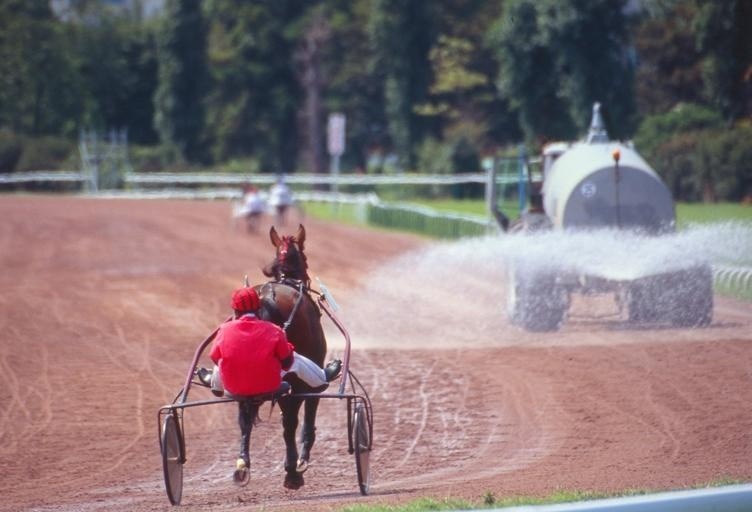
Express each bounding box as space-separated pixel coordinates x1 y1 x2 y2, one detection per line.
197 367 223 398
313 359 342 392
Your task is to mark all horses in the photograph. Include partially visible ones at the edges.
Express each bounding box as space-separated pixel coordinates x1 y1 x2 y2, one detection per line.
230 222 328 488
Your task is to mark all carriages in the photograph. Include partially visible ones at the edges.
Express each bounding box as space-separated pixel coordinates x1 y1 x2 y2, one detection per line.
157 224 373 506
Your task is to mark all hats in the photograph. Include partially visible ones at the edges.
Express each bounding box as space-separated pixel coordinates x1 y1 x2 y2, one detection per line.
230 288 262 311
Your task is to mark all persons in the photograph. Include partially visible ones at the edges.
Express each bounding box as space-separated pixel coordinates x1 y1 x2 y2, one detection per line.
234 185 267 218
265 176 294 211
193 285 343 402
